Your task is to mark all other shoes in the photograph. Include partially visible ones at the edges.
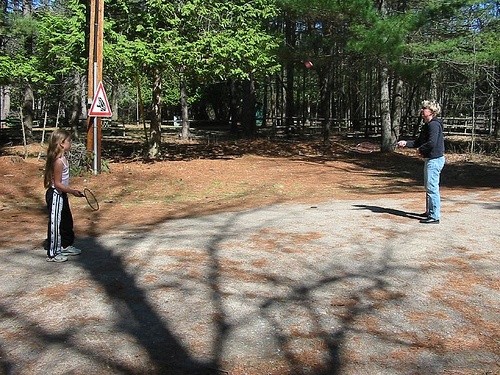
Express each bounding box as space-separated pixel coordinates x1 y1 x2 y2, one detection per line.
417 213 428 220
420 217 439 224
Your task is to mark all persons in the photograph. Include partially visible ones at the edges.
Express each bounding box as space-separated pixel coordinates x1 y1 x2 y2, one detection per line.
44 129 83 262
396 99 445 224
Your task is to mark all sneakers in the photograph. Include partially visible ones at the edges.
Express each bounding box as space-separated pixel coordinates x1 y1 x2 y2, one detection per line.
45 254 68 263
61 245 82 256
